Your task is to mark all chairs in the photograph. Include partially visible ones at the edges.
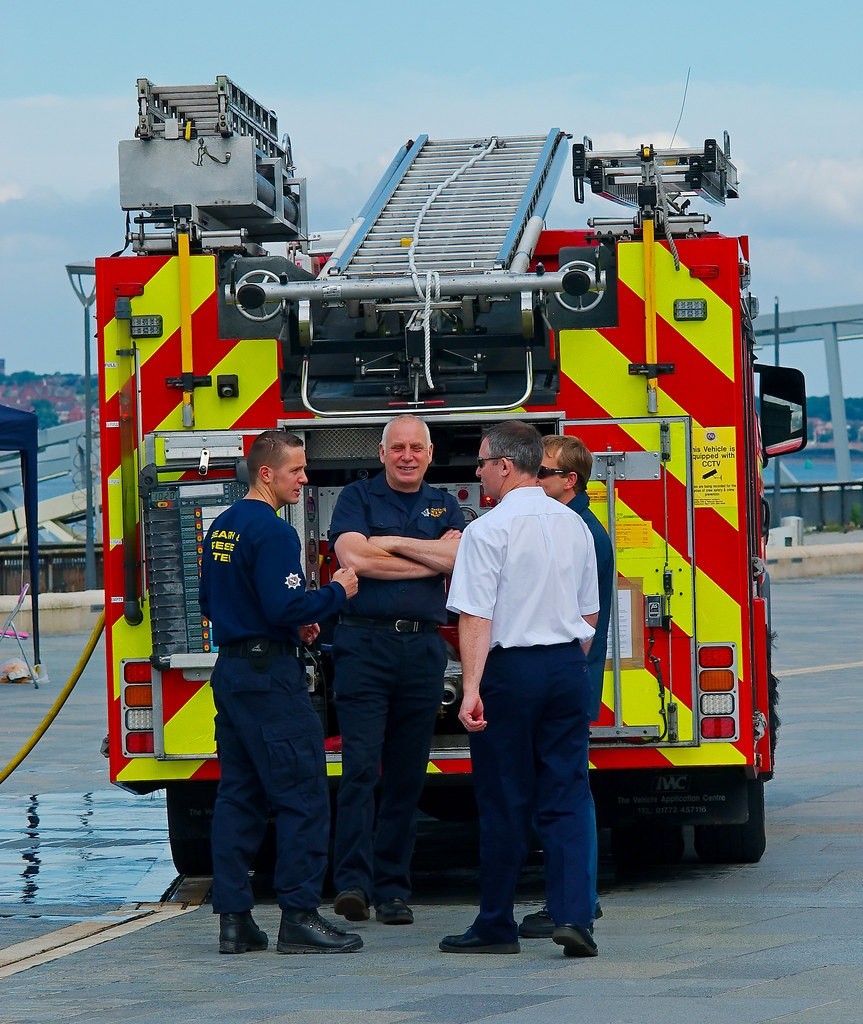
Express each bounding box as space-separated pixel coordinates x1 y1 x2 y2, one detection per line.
0 583 39 689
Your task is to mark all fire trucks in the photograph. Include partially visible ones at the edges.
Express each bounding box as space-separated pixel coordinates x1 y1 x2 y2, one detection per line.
94 76 809 880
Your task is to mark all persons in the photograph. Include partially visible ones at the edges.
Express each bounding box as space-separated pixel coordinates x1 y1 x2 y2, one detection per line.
439 420 600 958
515 434 614 939
198 431 364 953
326 414 468 925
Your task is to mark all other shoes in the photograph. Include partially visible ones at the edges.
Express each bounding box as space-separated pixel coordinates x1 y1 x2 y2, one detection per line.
522 902 603 921
518 912 594 938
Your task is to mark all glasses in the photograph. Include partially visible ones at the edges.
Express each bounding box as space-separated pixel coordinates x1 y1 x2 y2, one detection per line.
537 466 580 480
476 456 514 469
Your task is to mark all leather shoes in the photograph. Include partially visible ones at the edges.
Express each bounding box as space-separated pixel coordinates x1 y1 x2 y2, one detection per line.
276 908 363 954
375 898 414 923
219 910 268 953
334 886 370 921
552 923 598 957
439 925 520 954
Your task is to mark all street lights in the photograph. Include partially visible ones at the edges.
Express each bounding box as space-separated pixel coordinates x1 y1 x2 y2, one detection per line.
62 261 97 593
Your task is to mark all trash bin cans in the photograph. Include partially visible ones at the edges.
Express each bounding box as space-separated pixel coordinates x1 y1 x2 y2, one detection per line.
781 516 804 563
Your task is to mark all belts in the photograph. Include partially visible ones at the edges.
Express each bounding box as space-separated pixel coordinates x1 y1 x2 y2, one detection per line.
338 614 440 634
219 637 306 664
